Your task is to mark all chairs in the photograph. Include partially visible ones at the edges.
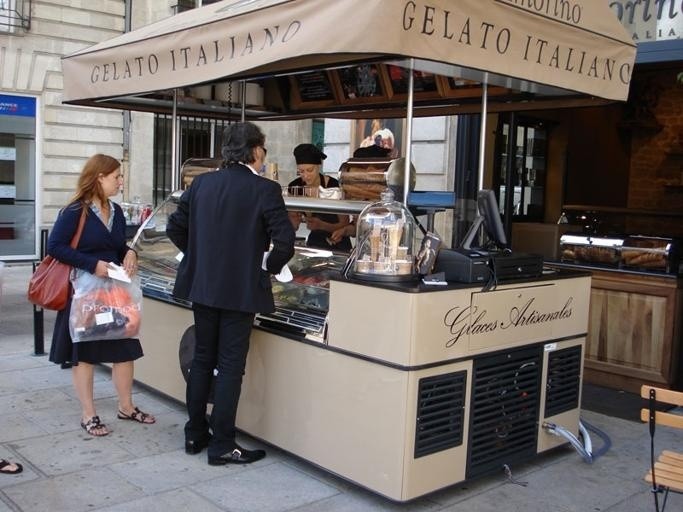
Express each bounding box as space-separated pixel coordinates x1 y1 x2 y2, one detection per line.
628 383 682 511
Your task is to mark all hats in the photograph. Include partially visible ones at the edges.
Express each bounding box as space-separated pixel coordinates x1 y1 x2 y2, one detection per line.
292 143 328 166
352 144 392 158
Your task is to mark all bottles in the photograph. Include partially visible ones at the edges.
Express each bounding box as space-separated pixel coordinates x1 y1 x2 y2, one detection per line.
119 195 156 230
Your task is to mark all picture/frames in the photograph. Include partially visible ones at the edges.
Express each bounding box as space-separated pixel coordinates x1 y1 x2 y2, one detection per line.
380 57 445 105
331 60 388 106
439 69 512 99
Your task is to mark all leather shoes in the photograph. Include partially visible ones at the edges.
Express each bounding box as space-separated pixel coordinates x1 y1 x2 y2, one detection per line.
184 430 215 455
206 440 267 467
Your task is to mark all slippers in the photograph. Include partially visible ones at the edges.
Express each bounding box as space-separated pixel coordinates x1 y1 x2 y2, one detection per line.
0 456 22 476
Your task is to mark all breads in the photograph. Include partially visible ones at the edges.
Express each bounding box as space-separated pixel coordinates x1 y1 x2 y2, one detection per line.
341 167 389 199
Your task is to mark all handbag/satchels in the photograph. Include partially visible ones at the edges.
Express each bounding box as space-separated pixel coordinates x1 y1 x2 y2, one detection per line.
26 252 77 313
63 256 147 346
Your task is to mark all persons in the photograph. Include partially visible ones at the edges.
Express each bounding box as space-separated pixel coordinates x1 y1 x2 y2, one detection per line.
416 238 435 274
286 143 353 252
0 458 23 474
165 121 294 466
331 145 393 244
45 154 157 436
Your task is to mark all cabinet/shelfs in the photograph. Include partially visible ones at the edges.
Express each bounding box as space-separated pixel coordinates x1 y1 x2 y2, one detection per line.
546 200 682 399
117 187 372 349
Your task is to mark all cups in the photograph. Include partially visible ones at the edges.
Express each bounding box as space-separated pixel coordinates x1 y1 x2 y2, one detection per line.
281 185 317 199
356 247 412 275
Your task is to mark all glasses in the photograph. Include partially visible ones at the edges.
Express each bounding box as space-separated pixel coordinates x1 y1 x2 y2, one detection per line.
260 146 268 156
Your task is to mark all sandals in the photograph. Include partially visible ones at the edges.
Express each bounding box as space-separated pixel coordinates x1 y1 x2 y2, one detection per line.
79 414 108 437
116 407 156 425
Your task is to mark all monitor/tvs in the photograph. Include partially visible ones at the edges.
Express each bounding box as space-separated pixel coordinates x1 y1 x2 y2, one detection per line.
476 190 508 249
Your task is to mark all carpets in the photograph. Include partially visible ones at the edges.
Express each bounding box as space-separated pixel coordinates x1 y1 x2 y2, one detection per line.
578 378 676 427
